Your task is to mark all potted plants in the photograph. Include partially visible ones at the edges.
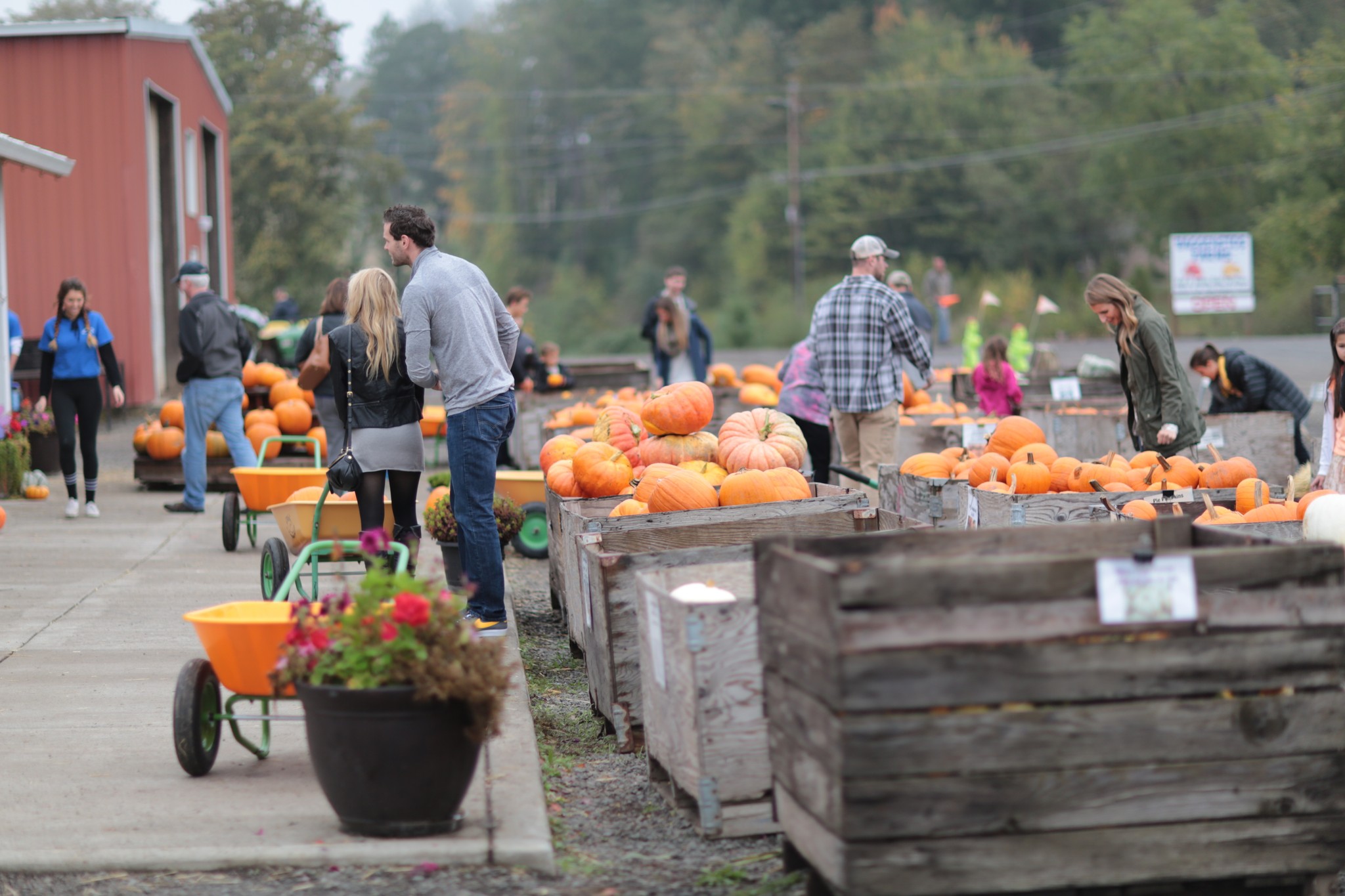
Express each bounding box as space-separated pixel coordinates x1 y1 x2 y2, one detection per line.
422 494 528 594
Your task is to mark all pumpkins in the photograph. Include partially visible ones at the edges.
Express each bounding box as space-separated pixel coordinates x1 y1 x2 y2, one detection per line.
518 373 818 517
709 362 1096 421
134 358 333 464
903 415 1345 547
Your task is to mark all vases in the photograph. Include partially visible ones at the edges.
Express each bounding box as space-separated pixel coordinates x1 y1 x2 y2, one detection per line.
27 426 65 477
289 668 495 839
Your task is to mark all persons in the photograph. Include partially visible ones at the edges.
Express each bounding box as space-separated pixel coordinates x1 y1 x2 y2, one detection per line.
380 204 518 638
922 256 958 344
885 267 935 348
1308 317 1345 495
34 278 125 517
640 265 700 366
970 338 1025 418
1083 273 1207 459
805 235 934 506
1190 341 1313 464
267 287 298 324
8 309 24 373
164 261 260 513
502 286 578 393
775 336 836 484
295 277 349 466
652 298 716 389
327 268 427 580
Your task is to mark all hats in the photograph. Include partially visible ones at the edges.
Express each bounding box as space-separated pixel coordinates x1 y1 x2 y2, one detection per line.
848 233 900 261
887 269 912 287
170 260 208 285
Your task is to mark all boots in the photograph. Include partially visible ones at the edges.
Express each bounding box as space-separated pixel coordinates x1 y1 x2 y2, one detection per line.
392 524 422 580
358 529 388 574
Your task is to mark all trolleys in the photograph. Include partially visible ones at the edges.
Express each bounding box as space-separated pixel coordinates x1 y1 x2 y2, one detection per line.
491 469 552 559
171 539 410 776
260 466 395 599
220 435 334 551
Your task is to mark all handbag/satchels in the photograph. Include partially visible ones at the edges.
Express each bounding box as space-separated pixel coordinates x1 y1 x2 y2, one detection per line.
297 315 330 391
325 450 363 497
937 292 960 308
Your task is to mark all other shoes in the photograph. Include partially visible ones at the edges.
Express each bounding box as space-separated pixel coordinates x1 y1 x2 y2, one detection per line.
178 509 179 510
84 501 100 517
65 497 80 517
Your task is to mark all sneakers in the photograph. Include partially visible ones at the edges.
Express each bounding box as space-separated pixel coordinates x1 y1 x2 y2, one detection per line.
453 608 508 637
165 502 203 512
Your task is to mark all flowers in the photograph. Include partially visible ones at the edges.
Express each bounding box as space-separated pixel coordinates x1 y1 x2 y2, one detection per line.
2 404 82 442
275 530 472 693
